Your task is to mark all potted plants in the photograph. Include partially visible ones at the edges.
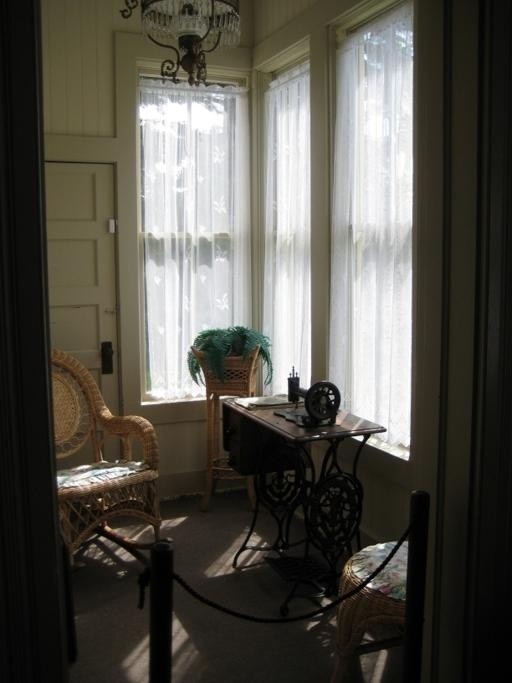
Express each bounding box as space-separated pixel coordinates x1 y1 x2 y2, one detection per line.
190 328 270 396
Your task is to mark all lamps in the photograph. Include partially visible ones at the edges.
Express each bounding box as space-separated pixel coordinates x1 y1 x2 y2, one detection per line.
119 0 243 87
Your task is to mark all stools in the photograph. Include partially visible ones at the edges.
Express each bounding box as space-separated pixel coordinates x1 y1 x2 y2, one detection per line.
329 537 409 683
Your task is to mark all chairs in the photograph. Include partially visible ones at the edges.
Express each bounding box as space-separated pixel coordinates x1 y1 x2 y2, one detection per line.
49 348 161 563
